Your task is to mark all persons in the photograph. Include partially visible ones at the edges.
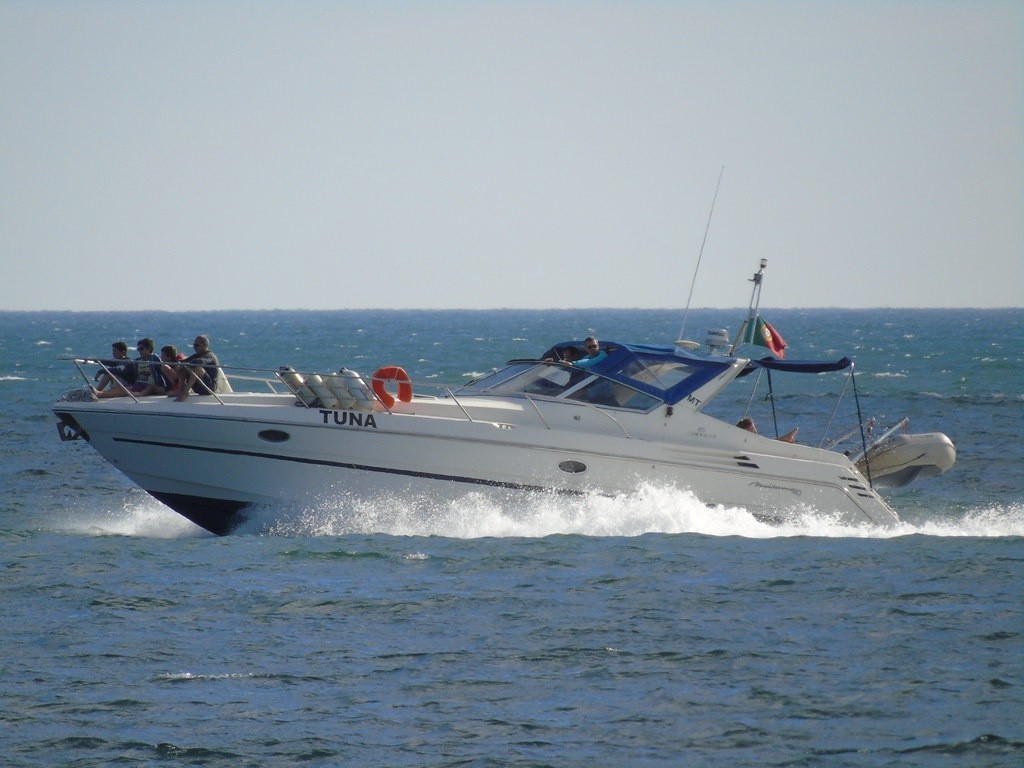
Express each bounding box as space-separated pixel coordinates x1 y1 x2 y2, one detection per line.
735 417 799 443
88 336 219 403
546 336 621 407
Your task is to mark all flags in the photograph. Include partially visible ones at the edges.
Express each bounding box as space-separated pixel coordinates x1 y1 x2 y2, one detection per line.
743 308 787 360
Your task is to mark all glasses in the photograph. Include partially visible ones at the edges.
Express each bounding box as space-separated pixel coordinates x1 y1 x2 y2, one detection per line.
193 343 203 347
588 345 597 349
137 346 149 351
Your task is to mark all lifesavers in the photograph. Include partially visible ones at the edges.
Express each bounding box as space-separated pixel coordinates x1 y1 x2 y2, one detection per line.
369 363 416 407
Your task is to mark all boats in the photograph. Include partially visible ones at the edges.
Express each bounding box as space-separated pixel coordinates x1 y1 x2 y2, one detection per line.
50 261 958 540
